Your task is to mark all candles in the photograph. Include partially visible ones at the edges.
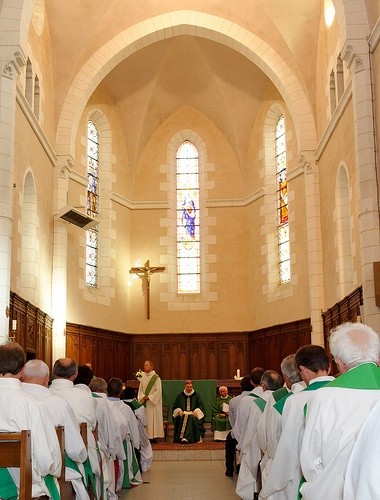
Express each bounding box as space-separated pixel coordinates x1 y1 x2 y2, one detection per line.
237 368 240 378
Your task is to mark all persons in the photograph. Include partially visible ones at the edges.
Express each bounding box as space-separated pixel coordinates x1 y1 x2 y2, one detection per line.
171 380 206 443
137 267 157 289
211 386 233 440
0 342 153 500
225 322 380 500
137 360 164 444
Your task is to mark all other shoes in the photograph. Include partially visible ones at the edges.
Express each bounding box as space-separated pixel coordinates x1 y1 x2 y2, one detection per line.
225 470 233 477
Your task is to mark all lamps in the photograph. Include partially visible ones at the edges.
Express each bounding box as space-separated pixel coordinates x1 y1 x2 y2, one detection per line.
59 206 99 230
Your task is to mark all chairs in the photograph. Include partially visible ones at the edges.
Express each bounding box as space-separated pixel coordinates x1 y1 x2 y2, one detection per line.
0 422 94 500
162 404 170 443
